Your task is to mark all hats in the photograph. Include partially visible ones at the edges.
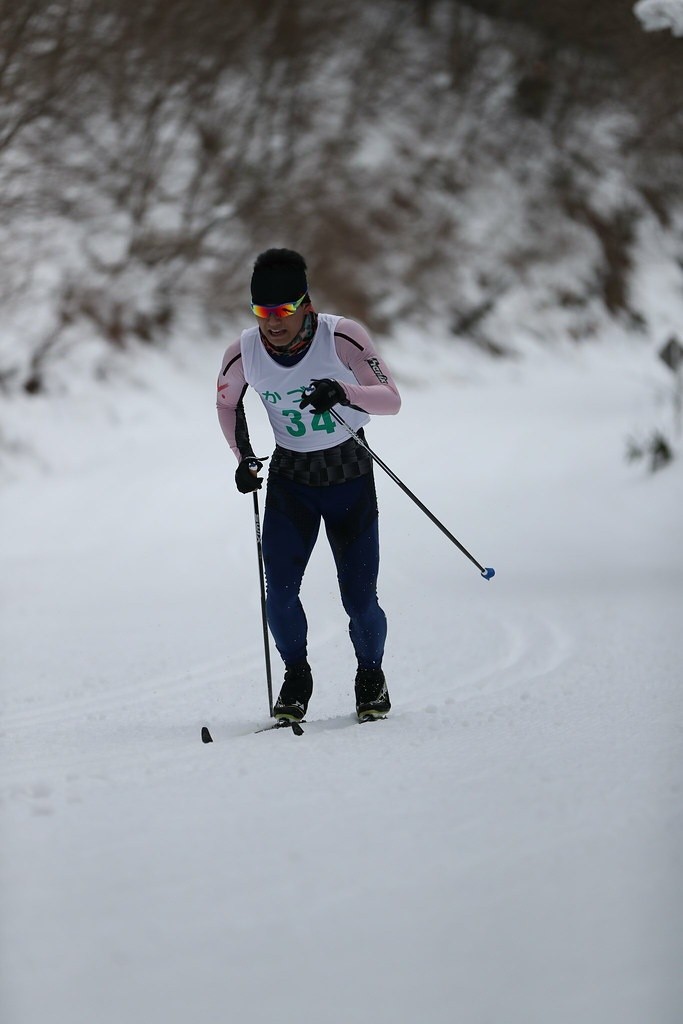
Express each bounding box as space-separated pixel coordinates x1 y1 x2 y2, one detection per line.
250 248 311 308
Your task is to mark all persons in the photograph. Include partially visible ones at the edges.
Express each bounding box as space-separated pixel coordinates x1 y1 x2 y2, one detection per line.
217 248 401 722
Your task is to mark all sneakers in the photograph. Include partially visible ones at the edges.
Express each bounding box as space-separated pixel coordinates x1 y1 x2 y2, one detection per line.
355 669 391 722
274 670 313 721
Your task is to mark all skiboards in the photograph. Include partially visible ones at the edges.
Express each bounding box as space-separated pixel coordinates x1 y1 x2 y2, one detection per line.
200 715 389 744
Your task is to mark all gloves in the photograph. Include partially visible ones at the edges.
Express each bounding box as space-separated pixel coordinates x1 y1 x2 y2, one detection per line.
235 458 264 494
298 378 347 416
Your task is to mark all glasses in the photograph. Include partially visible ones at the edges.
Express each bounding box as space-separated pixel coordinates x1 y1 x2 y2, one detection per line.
252 302 297 319
